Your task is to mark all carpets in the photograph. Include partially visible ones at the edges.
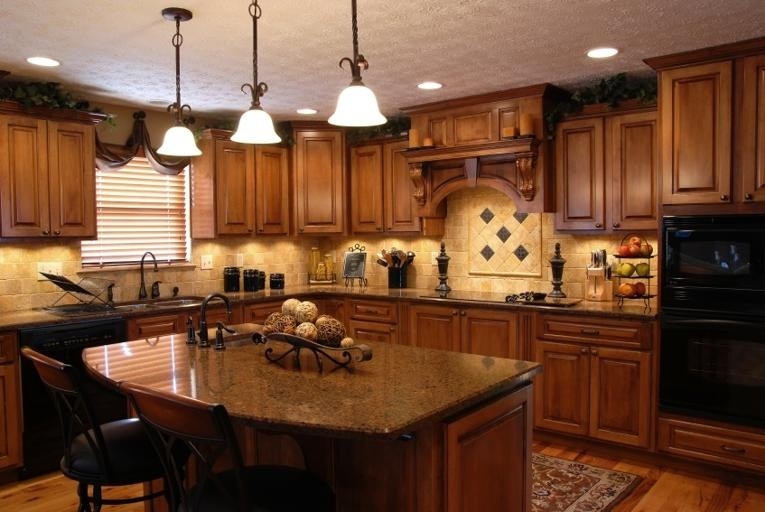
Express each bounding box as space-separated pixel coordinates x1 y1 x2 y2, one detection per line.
529 448 641 510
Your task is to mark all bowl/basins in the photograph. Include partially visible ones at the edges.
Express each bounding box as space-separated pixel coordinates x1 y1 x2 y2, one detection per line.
533 293 547 300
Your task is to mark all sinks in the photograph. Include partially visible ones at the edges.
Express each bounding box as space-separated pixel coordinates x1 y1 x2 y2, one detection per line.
209 332 262 346
114 297 206 314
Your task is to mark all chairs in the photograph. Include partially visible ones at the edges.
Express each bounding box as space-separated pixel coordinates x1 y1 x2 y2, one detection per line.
20 343 185 509
111 376 331 510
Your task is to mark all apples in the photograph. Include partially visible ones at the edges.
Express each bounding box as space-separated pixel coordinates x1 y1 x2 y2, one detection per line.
616 236 653 297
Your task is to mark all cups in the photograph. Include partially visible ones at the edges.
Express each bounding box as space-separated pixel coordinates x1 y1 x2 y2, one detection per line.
387 266 407 289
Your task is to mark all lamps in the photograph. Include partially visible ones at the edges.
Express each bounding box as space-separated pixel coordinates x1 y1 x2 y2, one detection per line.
158 6 205 161
227 3 282 149
325 0 386 128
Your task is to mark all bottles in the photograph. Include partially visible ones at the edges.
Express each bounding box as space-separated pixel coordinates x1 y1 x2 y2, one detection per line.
224 266 285 293
310 247 333 280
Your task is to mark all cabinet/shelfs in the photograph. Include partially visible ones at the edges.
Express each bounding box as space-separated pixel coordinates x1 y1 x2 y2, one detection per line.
329 294 399 343
0 100 95 240
350 139 425 237
533 309 658 452
405 88 551 146
131 295 325 419
556 103 656 233
655 60 764 215
190 131 287 240
658 414 765 474
406 304 530 363
290 123 350 238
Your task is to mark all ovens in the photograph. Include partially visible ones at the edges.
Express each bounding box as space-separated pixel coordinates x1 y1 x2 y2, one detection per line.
658 214 765 427
18 317 129 482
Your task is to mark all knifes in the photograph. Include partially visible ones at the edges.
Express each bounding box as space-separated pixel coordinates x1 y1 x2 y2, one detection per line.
590 248 607 267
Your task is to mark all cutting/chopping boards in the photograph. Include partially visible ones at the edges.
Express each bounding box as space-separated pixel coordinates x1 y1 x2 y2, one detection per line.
523 297 583 307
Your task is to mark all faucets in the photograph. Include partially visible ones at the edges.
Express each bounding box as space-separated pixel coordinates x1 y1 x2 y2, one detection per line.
197 292 233 346
137 251 159 299
